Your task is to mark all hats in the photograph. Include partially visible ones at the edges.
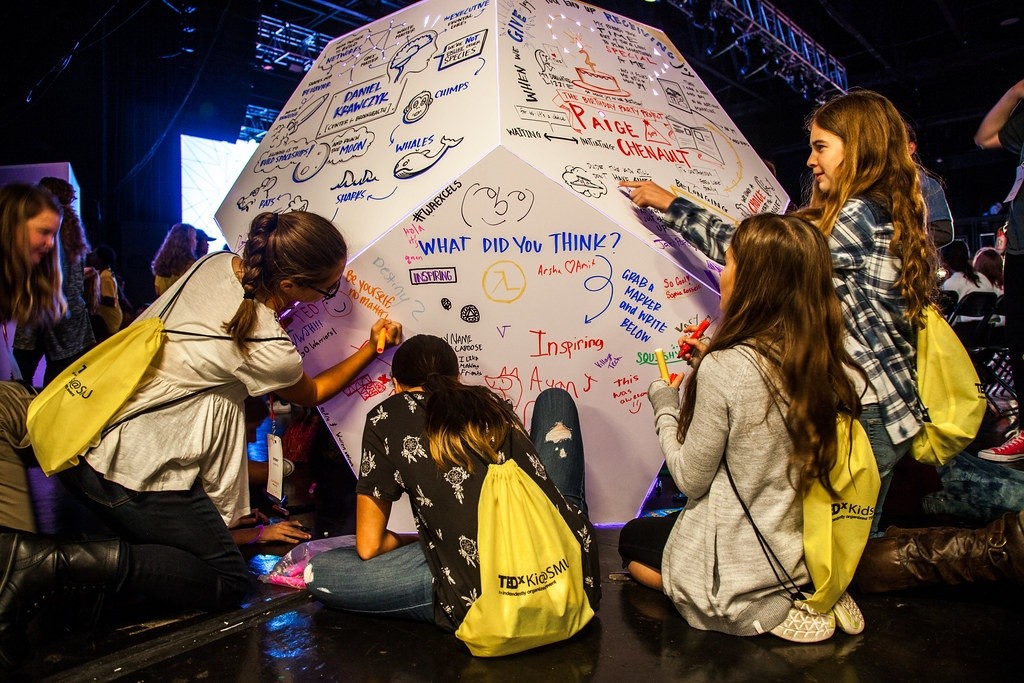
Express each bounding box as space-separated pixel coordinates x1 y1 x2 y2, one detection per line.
195 229 216 242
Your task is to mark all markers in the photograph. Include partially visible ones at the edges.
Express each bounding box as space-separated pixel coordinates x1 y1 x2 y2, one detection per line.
655 349 671 385
677 320 711 359
376 327 386 353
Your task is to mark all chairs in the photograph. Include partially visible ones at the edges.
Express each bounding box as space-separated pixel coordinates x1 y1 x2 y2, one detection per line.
934 290 1019 420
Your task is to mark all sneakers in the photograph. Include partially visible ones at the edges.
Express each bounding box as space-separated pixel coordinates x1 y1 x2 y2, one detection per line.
977 427 1024 462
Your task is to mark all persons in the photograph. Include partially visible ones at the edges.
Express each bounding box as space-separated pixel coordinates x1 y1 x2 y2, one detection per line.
619 90 941 538
618 213 867 642
0 177 232 539
900 118 955 250
929 231 1008 352
0 210 407 654
356 334 602 640
972 78 1024 461
224 393 341 546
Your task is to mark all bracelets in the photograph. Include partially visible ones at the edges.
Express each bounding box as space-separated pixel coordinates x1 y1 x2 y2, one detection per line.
249 524 265 545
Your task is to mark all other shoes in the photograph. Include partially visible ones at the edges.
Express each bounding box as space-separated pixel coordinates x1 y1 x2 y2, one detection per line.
831 589 866 634
767 591 836 643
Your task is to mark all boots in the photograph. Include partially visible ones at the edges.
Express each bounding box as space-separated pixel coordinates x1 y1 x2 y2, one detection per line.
0 526 120 651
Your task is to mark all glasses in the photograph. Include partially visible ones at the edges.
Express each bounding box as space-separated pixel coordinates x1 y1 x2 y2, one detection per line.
272 273 341 300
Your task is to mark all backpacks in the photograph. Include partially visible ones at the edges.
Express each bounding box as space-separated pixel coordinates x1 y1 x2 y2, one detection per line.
903 294 987 465
402 388 596 658
720 340 882 611
26 250 291 478
